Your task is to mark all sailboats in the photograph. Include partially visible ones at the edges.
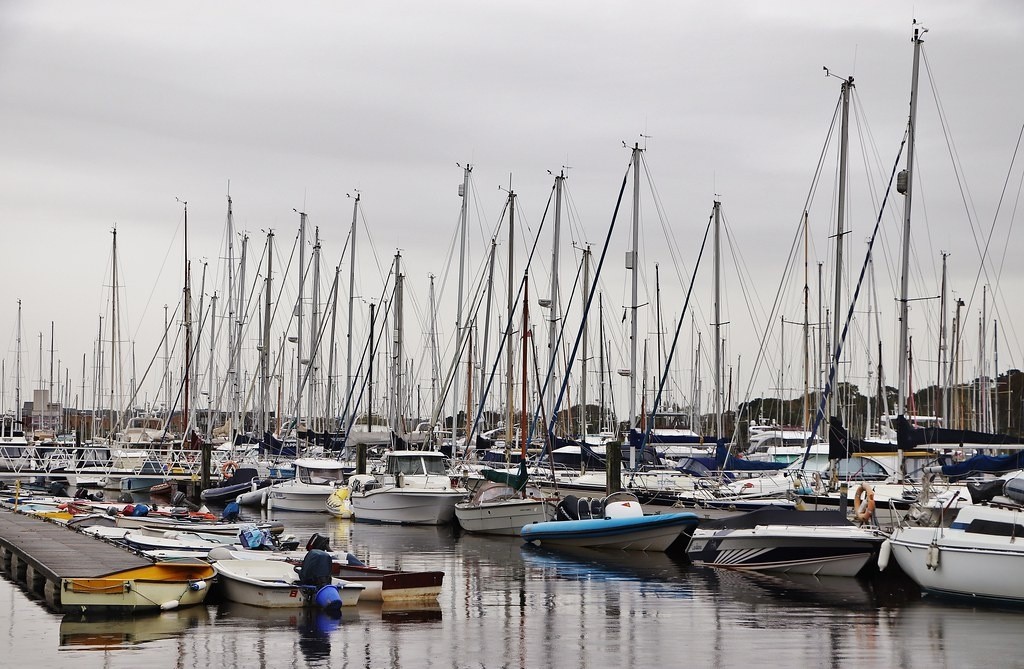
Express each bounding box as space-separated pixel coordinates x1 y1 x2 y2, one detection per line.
0 20 1024 607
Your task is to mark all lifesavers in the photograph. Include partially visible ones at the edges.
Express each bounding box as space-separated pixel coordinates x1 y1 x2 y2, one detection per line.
221 461 239 478
854 483 874 521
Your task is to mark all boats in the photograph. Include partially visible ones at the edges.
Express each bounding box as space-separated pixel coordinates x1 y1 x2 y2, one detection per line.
216 602 360 623
58 612 207 651
